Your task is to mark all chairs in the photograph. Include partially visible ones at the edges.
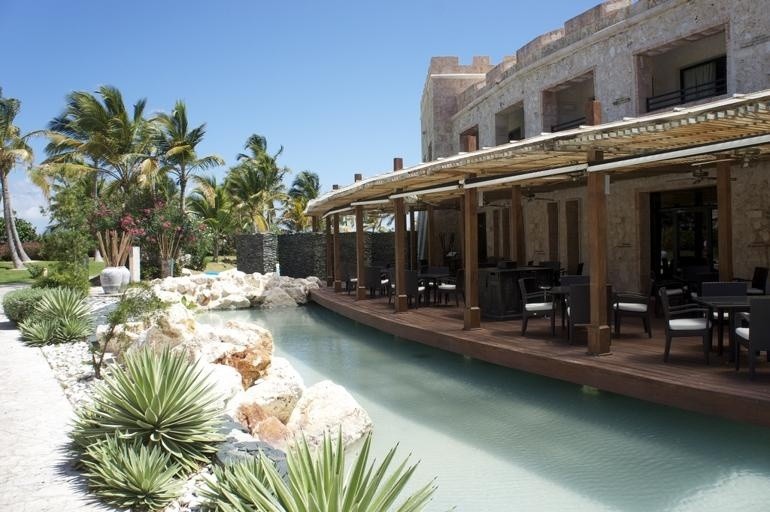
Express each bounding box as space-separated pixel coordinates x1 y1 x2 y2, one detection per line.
658 285 715 368
609 277 659 339
347 262 467 309
732 296 769 380
730 265 768 296
702 280 751 324
567 283 589 344
517 276 557 335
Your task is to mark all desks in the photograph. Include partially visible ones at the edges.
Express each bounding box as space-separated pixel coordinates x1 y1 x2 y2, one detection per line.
694 293 751 354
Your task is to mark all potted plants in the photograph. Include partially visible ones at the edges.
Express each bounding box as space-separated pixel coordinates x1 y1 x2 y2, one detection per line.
154 231 181 278
96 228 130 294
116 232 130 294
